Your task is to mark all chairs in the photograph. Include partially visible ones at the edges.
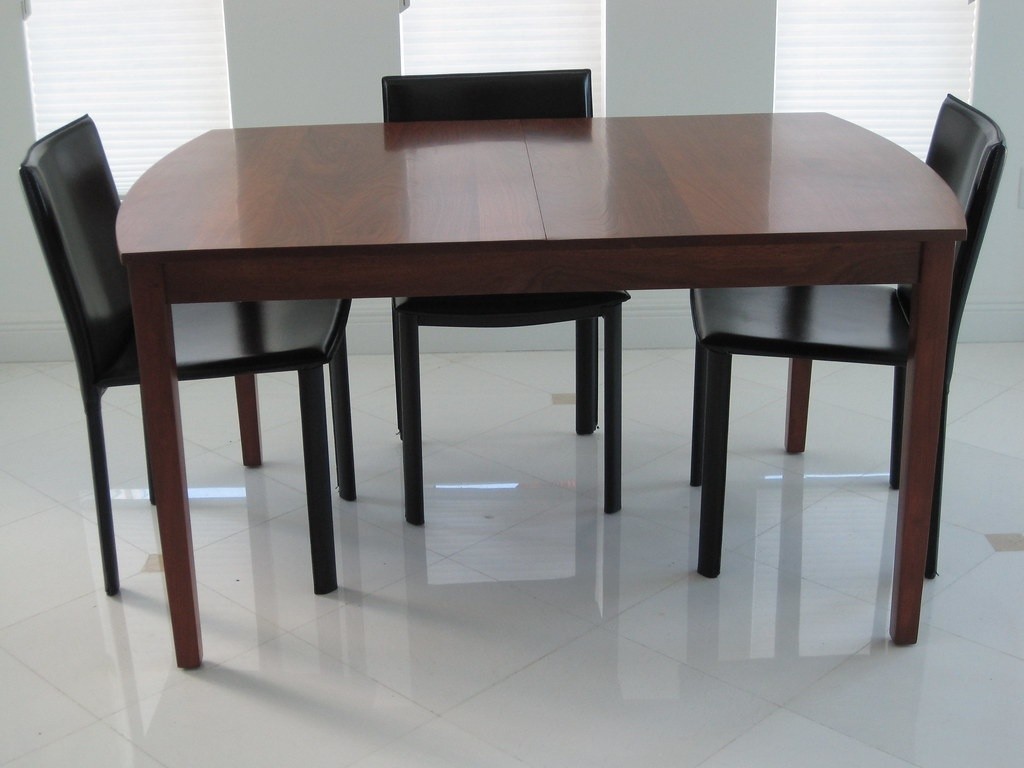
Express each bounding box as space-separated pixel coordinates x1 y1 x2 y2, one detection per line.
690 94 1006 577
381 69 634 527
17 112 358 596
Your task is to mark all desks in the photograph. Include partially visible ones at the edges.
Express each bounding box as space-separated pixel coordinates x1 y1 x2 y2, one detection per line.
115 111 967 673
